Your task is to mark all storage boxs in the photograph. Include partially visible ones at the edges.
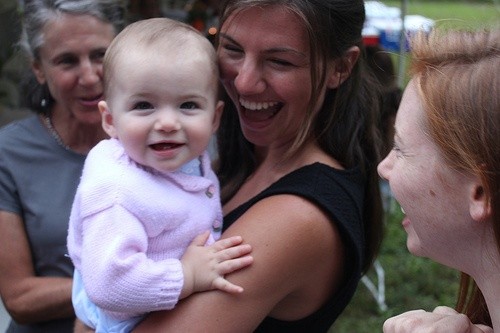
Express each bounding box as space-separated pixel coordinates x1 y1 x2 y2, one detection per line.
360 1 435 53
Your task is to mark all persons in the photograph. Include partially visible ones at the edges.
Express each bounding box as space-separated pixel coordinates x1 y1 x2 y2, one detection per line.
377 25 500 333
0 0 127 333
66 18 253 333
74 0 386 333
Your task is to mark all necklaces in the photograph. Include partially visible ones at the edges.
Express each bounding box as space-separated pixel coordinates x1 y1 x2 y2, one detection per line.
45 115 89 157
